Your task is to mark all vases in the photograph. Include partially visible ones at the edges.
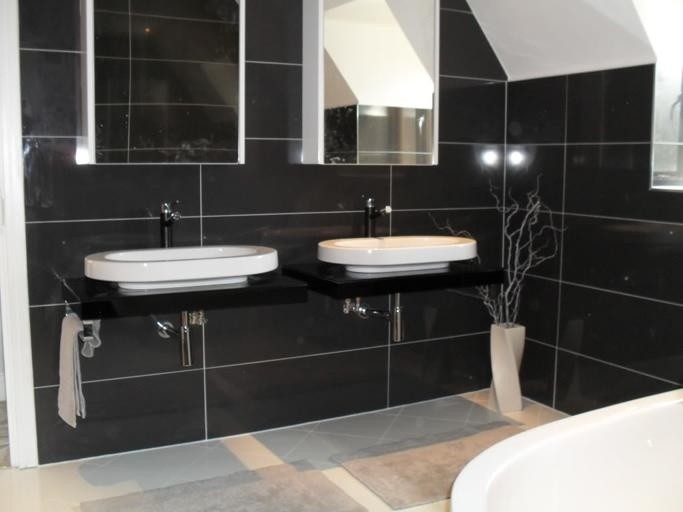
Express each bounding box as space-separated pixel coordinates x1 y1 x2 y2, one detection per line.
487 321 526 415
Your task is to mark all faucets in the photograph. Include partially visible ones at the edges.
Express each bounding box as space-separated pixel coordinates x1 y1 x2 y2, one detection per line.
159 198 183 248
361 192 393 237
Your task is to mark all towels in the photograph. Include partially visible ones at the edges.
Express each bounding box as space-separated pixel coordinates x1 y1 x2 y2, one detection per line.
57 312 90 431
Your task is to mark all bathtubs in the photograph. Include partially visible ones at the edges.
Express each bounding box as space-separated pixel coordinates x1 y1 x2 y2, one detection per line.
448 388 683 512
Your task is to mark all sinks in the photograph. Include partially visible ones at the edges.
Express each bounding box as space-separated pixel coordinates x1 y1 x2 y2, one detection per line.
316 234 477 273
83 246 280 289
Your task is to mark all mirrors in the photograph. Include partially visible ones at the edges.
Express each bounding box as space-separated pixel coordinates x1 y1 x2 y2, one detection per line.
319 1 439 164
90 0 242 165
